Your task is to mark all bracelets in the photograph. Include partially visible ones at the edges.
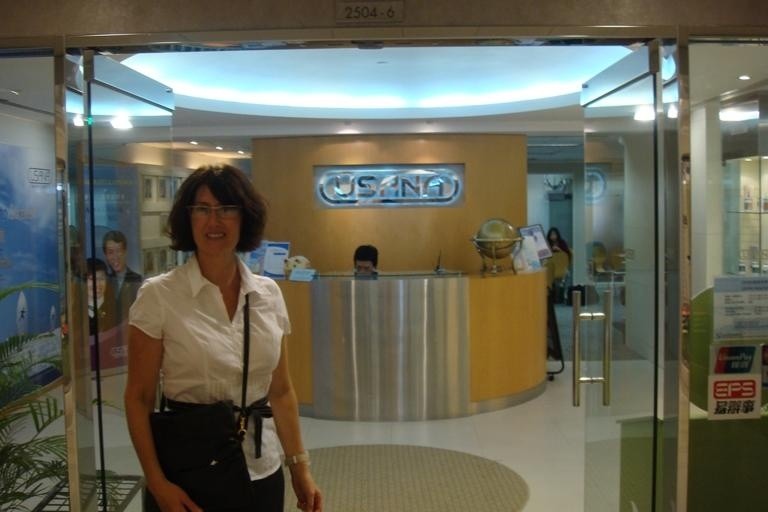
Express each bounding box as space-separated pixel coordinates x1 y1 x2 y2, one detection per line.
284 451 311 467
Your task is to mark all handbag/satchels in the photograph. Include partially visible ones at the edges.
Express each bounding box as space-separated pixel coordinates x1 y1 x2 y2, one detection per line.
149 400 258 512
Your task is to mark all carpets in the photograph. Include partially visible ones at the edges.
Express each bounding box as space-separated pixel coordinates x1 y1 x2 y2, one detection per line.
275 446 530 512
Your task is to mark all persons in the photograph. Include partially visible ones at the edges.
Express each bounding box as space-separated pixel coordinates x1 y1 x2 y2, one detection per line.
125 164 322 512
103 231 143 325
354 244 377 274
548 227 561 251
87 257 118 336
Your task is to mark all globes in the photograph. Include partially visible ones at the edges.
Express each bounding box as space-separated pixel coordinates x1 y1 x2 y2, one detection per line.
472 218 524 277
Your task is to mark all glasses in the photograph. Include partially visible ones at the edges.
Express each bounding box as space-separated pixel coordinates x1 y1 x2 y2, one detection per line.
190 203 243 220
